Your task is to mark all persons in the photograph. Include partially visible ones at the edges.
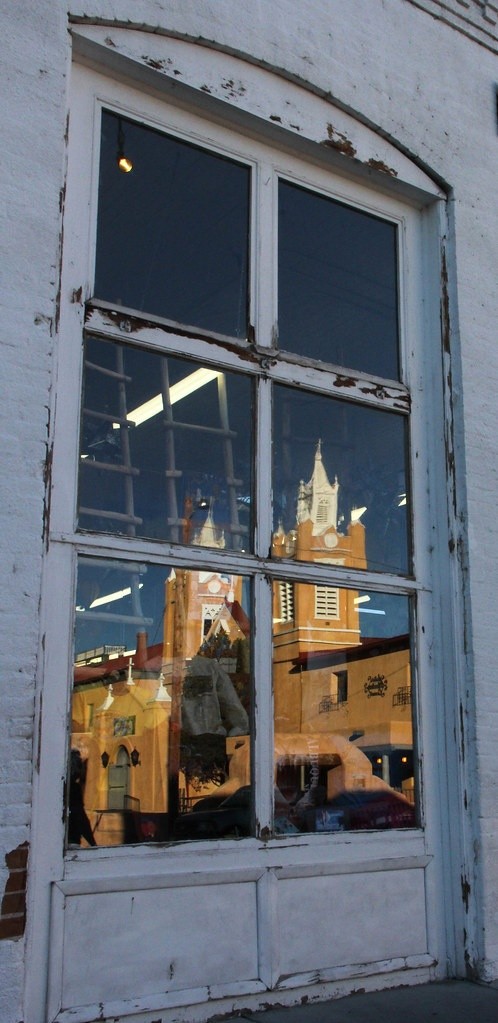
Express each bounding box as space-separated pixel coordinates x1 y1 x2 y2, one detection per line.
179 646 246 782
273 765 307 833
74 666 117 681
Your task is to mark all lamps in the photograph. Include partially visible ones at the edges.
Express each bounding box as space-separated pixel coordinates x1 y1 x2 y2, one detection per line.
88 582 144 608
116 120 131 179
113 368 224 431
